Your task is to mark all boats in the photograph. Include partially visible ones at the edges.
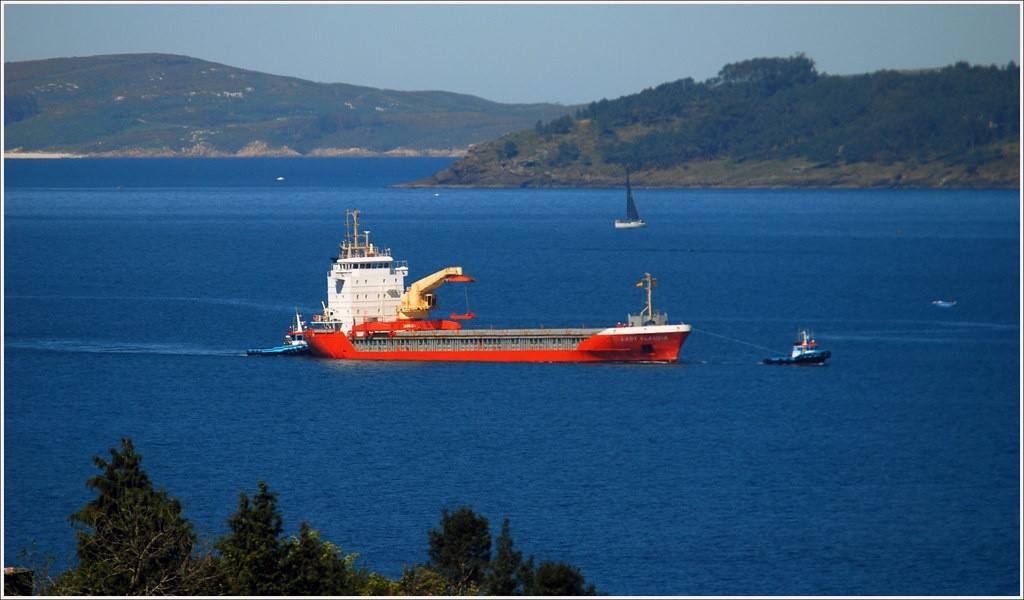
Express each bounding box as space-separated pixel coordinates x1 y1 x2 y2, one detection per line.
246 330 309 356
285 209 692 365
765 328 831 365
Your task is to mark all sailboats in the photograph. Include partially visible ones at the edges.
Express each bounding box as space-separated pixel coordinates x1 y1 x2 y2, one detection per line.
613 169 646 228
